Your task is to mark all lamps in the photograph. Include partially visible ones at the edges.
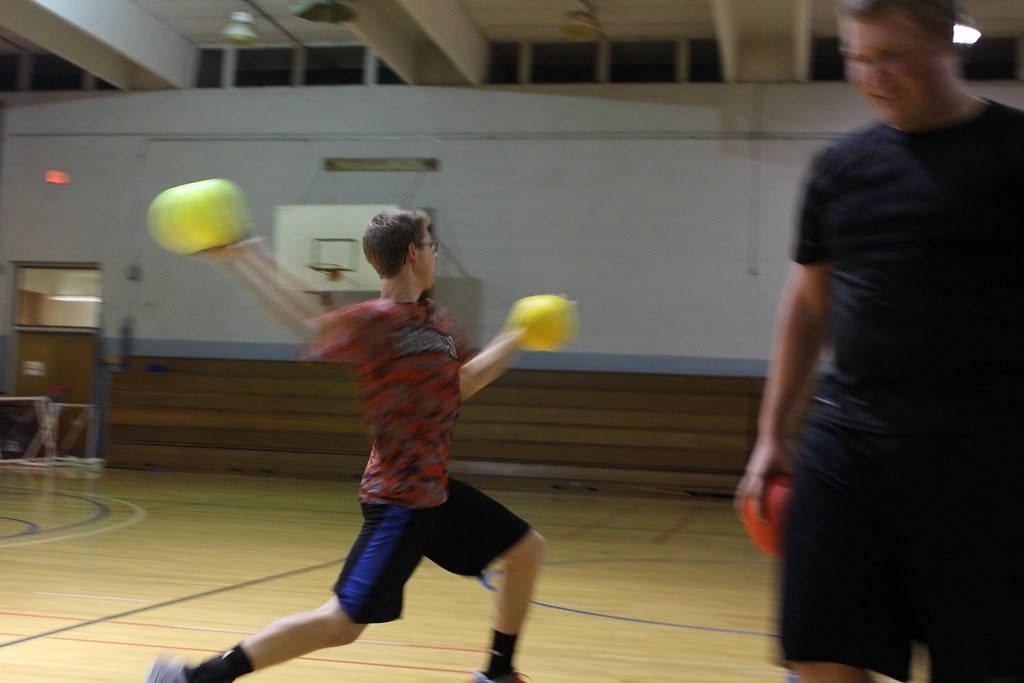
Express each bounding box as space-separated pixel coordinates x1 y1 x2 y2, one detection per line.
286 0 358 23
218 1 263 46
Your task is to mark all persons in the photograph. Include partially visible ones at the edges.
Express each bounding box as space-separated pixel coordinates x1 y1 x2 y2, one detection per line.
150 211 547 683
740 0 1024 682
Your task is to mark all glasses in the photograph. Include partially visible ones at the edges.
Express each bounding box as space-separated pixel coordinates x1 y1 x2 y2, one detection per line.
403 241 439 257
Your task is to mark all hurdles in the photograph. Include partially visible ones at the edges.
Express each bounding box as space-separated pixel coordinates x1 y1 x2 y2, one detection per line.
0 396 106 467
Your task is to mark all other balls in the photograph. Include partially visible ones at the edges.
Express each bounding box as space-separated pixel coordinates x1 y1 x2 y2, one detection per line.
143 178 256 263
736 480 793 559
507 293 581 354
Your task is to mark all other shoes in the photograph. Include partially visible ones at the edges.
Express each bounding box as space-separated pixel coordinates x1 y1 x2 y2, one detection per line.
471 669 534 683
144 655 189 683
